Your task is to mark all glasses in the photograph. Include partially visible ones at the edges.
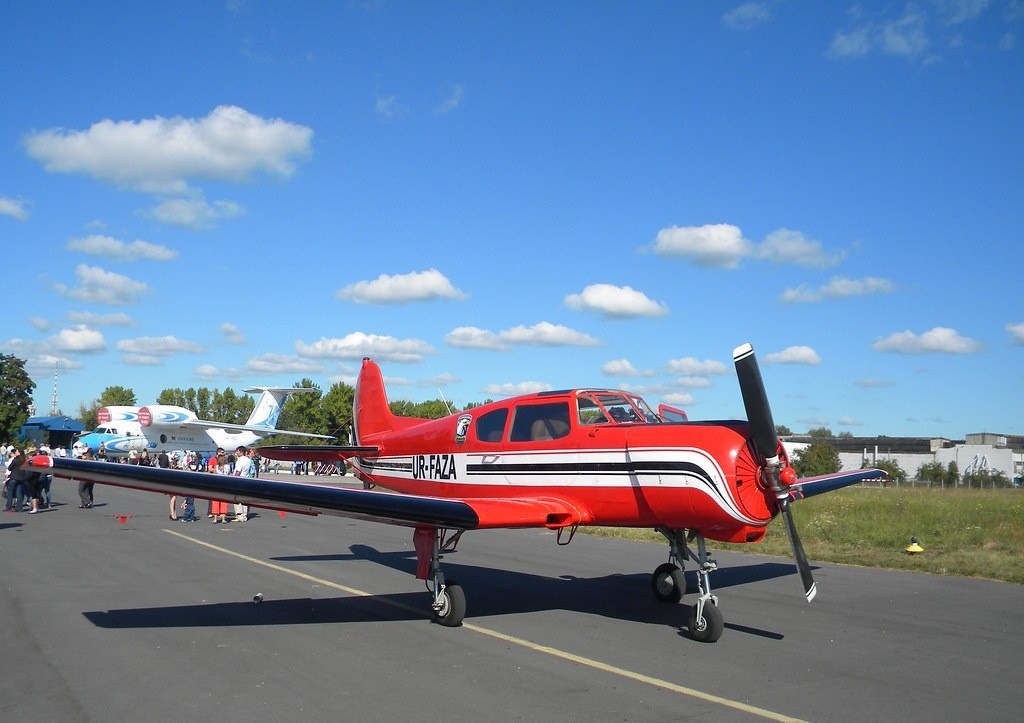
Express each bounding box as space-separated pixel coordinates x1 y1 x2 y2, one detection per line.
219 452 226 454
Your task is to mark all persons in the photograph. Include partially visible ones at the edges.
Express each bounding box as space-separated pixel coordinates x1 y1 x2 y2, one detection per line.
180 497 195 522
168 465 180 521
2 448 54 513
211 455 231 524
273 460 280 474
231 446 252 522
78 452 93 508
245 450 255 478
83 446 94 503
290 464 294 475
263 458 270 473
248 448 262 479
0 442 211 471
295 460 319 475
233 453 238 470
228 454 234 473
207 447 227 518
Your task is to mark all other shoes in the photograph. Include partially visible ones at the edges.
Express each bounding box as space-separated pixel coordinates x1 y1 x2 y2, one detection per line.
3 508 11 512
79 502 92 508
181 519 188 522
222 520 228 524
169 515 179 521
191 519 195 523
28 509 38 514
16 508 23 512
213 520 218 524
231 516 247 522
208 514 214 518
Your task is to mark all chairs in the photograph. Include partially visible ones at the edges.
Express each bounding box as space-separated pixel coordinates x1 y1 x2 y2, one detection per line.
531 419 570 442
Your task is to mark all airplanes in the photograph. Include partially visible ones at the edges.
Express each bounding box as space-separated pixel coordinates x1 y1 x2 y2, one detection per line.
66 386 335 462
16 342 892 641
